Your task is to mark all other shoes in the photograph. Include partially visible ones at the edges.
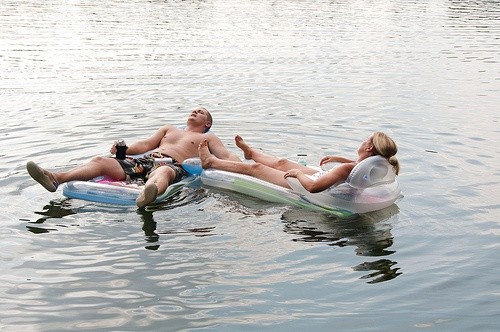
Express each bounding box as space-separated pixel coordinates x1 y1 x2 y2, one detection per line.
26 161 59 192
136 183 158 209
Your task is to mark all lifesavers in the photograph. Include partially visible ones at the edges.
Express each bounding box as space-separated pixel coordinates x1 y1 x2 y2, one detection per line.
62 157 401 216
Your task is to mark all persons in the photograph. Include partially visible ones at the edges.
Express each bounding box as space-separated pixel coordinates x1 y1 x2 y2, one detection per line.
26 107 243 209
198 131 397 193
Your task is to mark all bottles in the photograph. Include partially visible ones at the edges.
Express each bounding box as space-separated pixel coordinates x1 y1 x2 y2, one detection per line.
114 138 127 159
298 157 307 166
